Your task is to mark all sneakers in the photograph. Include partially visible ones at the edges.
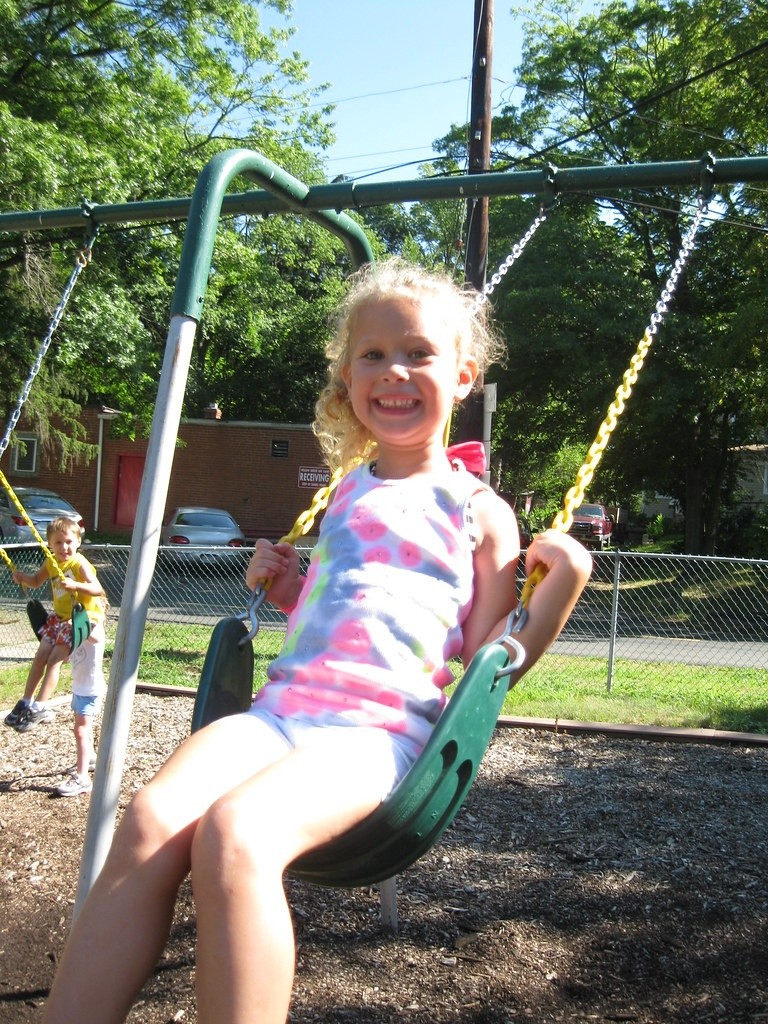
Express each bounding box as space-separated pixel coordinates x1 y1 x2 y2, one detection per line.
55 775 93 795
5 699 25 724
67 756 96 773
15 701 56 733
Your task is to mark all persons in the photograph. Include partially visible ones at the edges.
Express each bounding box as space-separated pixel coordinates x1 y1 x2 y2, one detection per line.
5 517 103 732
38 258 593 1023
55 595 110 796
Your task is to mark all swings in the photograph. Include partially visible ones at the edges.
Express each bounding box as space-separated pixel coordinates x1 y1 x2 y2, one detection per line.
192 185 720 886
0 226 104 656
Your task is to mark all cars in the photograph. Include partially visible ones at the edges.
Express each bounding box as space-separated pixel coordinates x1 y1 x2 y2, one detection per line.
568 503 612 550
162 505 248 571
0 487 86 545
